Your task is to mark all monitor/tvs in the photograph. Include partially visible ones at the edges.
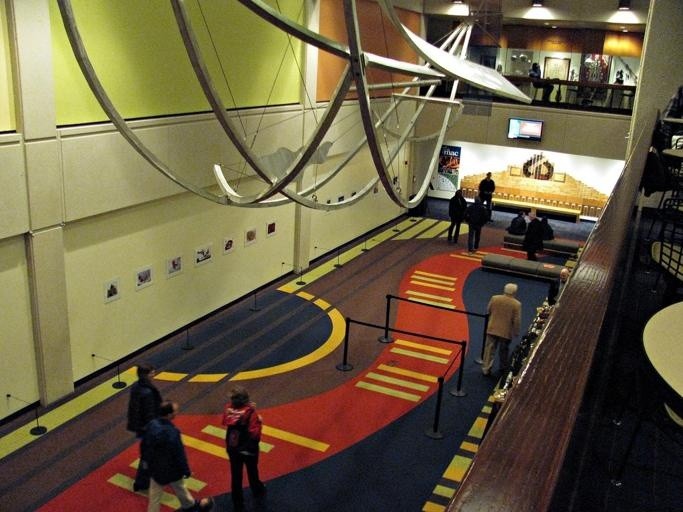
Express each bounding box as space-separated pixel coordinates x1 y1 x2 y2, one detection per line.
507 117 544 142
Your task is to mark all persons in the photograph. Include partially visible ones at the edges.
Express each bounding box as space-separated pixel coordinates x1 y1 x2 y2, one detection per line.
140 401 196 511
482 283 522 376
447 171 554 261
199 495 215 512
529 62 554 101
222 385 267 504
128 363 178 491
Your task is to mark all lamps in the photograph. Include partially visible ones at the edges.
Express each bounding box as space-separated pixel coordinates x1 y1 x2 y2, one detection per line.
447 0 641 25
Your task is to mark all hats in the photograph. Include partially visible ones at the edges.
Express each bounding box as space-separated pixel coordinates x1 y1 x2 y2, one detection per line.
504 283 518 294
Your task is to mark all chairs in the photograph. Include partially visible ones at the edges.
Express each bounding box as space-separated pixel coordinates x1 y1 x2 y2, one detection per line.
614 109 683 308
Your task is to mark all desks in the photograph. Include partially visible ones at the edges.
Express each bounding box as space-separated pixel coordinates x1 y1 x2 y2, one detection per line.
611 301 683 486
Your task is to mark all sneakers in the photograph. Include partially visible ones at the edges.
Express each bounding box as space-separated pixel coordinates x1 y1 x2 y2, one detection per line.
483 370 490 375
233 502 246 509
447 237 459 242
468 240 478 252
254 484 267 497
133 480 149 491
183 498 213 511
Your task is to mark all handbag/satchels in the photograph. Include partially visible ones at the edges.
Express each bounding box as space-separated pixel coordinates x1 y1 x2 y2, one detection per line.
238 431 258 457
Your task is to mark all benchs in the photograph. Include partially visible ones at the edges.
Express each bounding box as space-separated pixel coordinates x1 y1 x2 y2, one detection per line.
481 254 566 282
503 232 580 258
